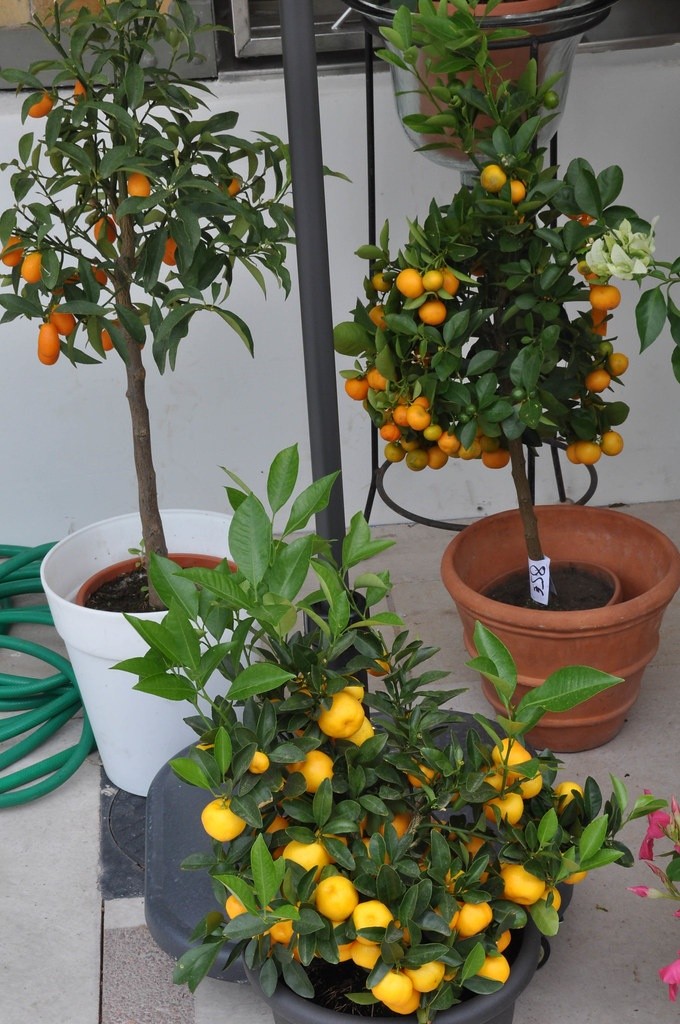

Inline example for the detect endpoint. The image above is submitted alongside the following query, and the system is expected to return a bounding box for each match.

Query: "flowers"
[335,0,680,600]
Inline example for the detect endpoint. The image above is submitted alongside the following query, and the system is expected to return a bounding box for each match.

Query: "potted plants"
[107,441,668,1024]
[0,0,238,614]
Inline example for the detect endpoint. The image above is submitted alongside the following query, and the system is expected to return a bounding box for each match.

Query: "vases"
[479,561,622,606]
[418,0,560,159]
[40,509,264,797]
[439,505,680,753]
